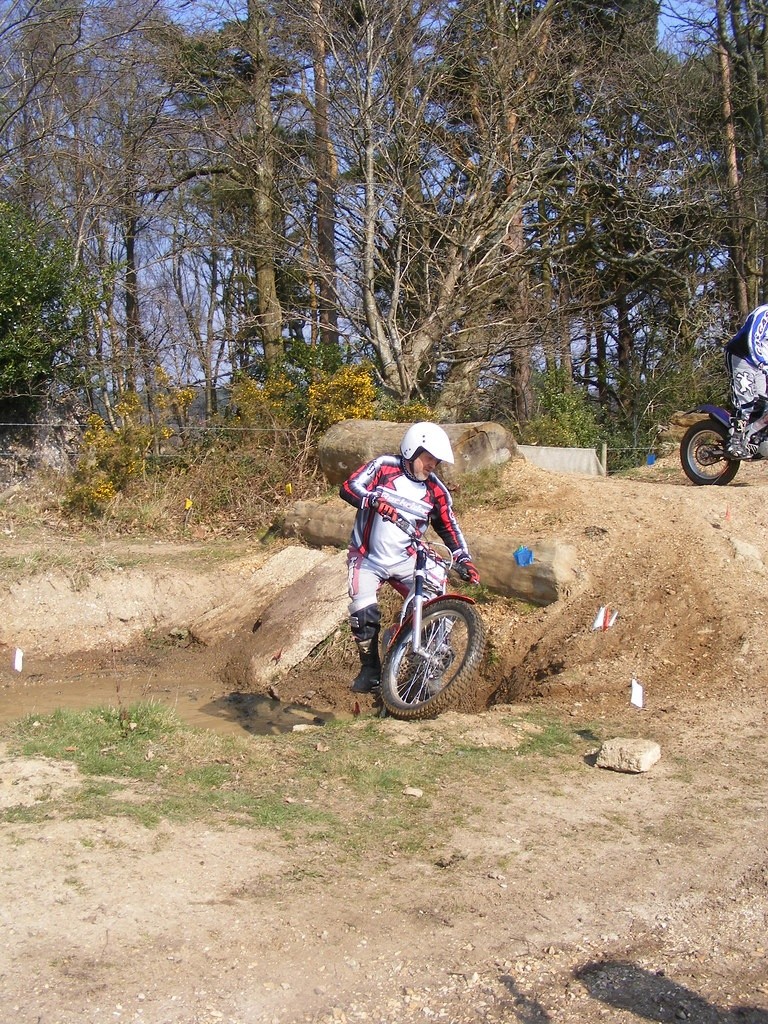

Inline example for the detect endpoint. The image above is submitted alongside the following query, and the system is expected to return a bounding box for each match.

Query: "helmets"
[401,422,457,464]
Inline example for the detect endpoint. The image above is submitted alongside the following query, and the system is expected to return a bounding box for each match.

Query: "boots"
[347,597,381,692]
[428,624,456,672]
[726,419,753,458]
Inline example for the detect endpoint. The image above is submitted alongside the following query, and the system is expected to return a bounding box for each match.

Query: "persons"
[724,304,768,459]
[340,421,480,693]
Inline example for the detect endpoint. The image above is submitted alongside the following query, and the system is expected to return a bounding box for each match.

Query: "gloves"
[376,496,397,522]
[458,562,480,584]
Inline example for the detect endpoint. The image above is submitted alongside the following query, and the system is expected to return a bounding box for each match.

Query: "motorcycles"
[368,494,487,722]
[680,404,768,486]
